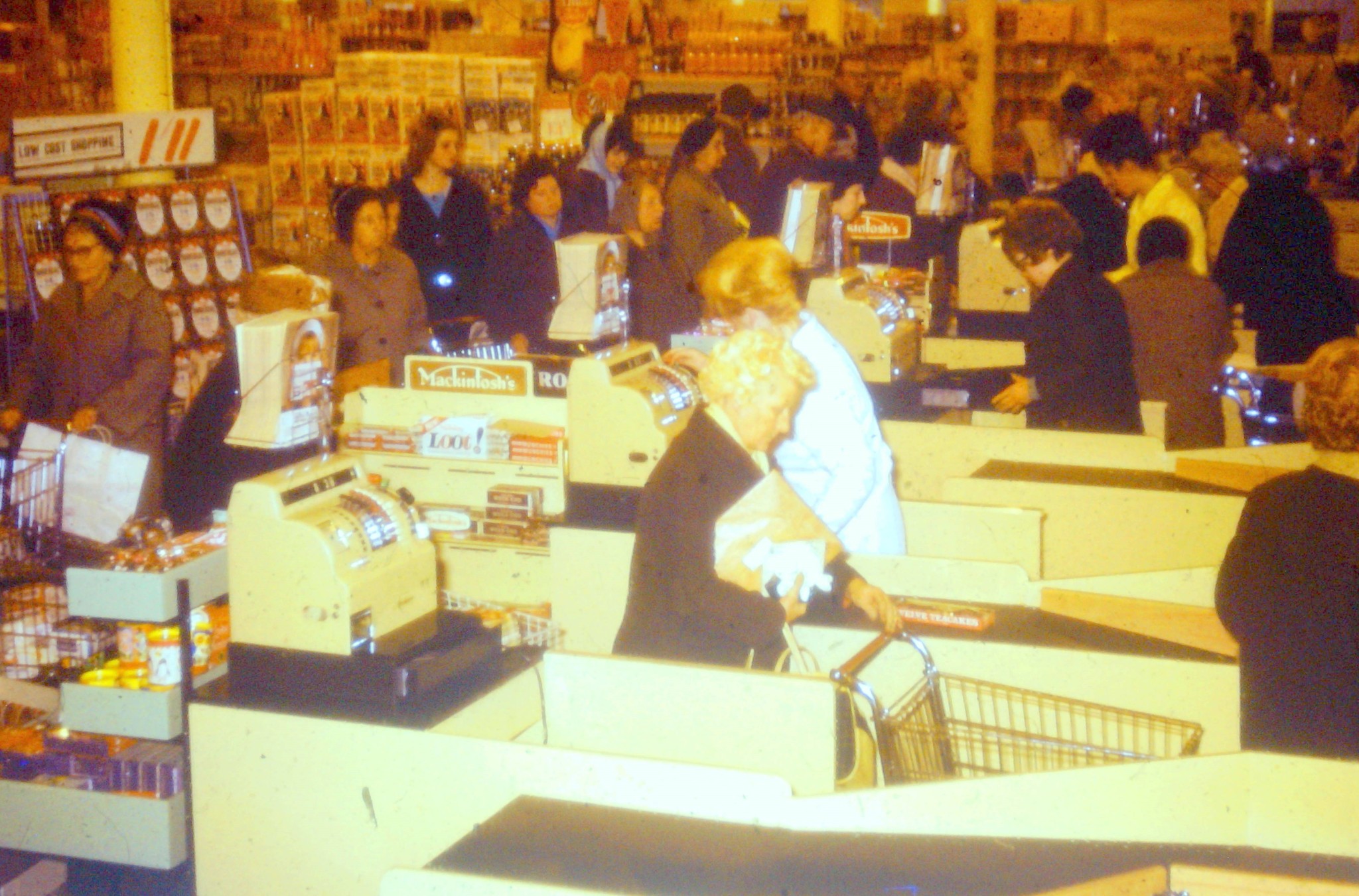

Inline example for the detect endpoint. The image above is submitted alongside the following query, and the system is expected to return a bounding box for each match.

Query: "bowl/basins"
[79,658,148,688]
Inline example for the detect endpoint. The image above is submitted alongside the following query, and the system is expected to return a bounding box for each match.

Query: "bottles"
[501,141,583,213]
[117,597,230,691]
[623,91,717,135]
[684,34,783,74]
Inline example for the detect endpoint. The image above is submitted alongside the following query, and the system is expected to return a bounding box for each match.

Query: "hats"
[800,92,850,139]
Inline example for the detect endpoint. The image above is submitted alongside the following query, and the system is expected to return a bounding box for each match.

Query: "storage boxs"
[477,485,541,541]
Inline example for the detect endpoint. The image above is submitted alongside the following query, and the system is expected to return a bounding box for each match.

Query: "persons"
[612,330,902,675]
[0,196,173,518]
[661,237,904,554]
[301,30,1359,453]
[1212,335,1359,763]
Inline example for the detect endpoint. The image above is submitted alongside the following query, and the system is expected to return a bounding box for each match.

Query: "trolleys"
[1210,366,1314,447]
[830,621,1207,788]
[1,422,76,578]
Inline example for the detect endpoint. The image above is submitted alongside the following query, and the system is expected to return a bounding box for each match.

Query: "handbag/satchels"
[11,422,149,545]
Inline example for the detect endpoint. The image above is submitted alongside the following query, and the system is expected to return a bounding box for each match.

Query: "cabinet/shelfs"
[1,0,1232,316]
[0,530,233,870]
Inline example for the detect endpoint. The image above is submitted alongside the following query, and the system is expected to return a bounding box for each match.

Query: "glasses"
[1012,249,1032,273]
[60,242,107,259]
